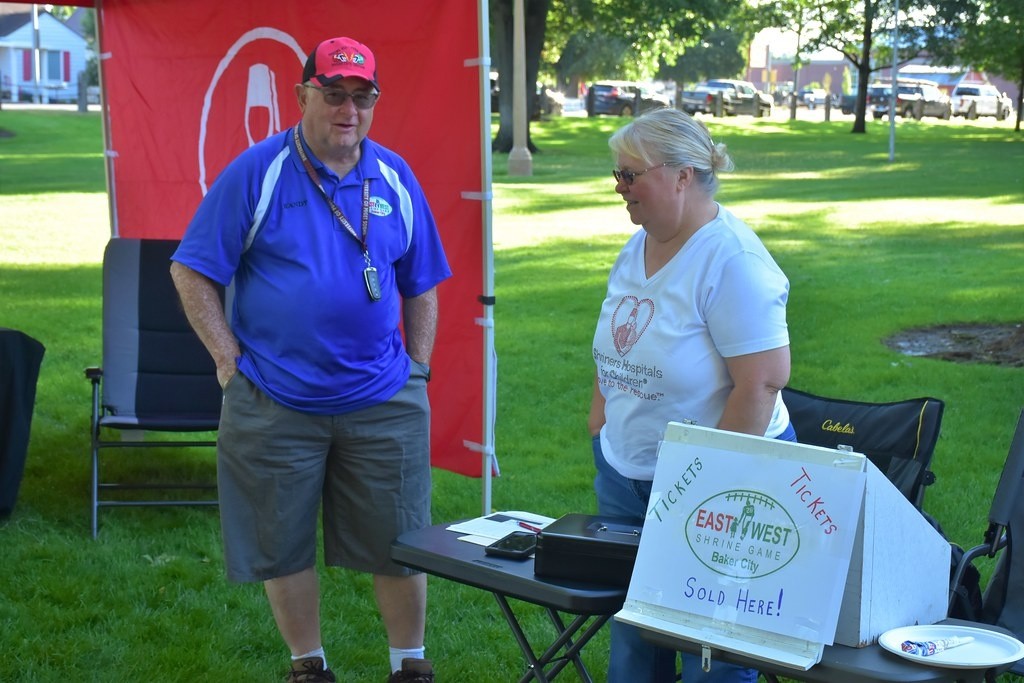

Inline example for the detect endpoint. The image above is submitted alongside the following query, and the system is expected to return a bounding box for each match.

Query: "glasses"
[612,161,668,186]
[301,82,382,109]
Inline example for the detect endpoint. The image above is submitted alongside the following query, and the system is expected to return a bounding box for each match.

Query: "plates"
[877,625,1024,671]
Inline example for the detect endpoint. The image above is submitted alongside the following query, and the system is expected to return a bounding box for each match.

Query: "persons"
[589,107,799,683]
[169,36,454,683]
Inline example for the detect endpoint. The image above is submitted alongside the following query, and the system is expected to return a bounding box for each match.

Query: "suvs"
[952,83,1014,120]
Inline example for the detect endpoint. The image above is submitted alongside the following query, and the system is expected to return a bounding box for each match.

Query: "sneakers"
[288,656,338,682]
[389,657,436,683]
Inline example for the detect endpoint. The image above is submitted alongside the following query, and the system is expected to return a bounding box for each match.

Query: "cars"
[584,80,666,116]
[669,78,951,120]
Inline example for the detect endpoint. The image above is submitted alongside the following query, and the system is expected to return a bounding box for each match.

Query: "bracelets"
[415,362,430,379]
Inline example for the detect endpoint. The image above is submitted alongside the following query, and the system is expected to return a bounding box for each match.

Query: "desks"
[390,513,630,683]
[644,617,1018,683]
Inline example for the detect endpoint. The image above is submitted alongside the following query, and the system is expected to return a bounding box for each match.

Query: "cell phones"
[485,530,538,561]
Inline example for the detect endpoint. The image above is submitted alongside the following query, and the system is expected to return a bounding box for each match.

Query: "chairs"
[781,385,946,508]
[82,236,235,539]
[947,407,1023,676]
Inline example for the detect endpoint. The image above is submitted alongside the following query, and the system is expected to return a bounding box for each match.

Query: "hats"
[300,37,381,92]
